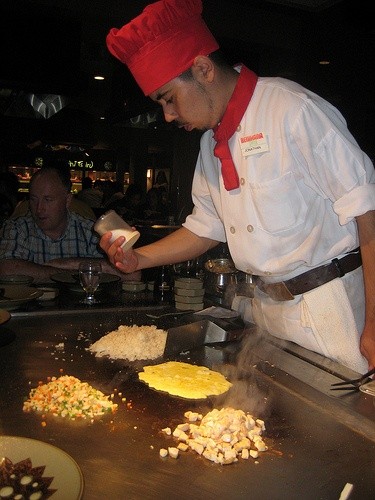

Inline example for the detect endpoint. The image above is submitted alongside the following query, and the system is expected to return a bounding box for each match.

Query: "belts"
[256,247,363,303]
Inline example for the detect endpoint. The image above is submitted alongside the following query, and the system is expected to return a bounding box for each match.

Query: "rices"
[52,325,168,362]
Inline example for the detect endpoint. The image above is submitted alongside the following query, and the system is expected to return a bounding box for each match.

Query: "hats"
[106,0,218,97]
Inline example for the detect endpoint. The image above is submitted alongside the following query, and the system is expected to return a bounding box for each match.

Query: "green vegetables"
[29,384,112,419]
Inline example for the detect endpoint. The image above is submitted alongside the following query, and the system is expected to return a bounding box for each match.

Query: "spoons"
[145,310,194,319]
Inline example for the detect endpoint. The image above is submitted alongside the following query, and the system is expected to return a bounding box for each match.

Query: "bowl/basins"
[203,258,259,296]
[0,270,121,325]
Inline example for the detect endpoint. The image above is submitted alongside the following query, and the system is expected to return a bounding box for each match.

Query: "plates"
[123,281,155,291]
[0,436,84,500]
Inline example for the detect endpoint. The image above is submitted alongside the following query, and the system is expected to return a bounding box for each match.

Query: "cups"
[94,209,140,254]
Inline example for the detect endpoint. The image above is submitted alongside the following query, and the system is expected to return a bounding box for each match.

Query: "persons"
[0,169,141,283]
[147,171,170,206]
[99,180,152,220]
[99,0,375,377]
[78,177,102,204]
[11,164,94,219]
[0,172,27,216]
[168,153,199,223]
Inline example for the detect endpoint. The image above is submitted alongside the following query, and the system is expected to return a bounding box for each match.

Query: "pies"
[138,360,232,399]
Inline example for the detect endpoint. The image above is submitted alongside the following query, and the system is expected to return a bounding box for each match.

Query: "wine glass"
[78,259,102,304]
